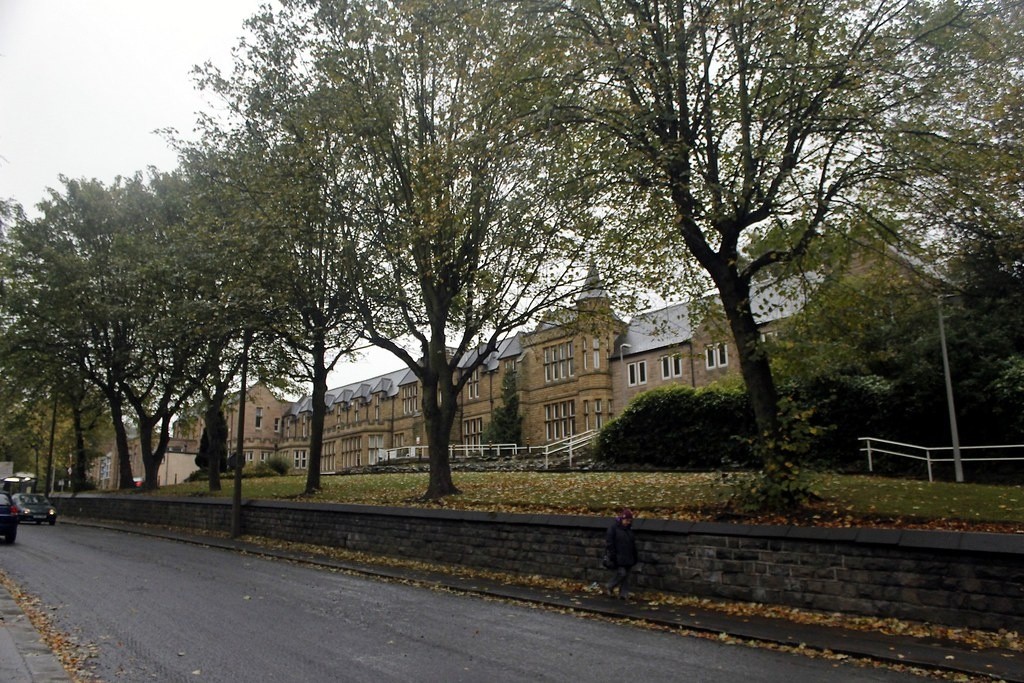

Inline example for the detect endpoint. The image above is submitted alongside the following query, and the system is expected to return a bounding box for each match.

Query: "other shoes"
[605,583,616,597]
[619,592,636,599]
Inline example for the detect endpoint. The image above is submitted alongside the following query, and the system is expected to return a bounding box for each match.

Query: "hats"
[622,509,633,518]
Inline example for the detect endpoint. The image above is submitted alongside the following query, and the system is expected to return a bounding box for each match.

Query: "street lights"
[231,324,255,540]
[937,294,964,482]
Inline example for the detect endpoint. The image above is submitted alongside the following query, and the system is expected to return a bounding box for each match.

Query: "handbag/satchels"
[603,556,614,569]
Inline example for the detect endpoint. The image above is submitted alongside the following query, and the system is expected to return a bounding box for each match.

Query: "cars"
[0,490,20,544]
[11,493,56,525]
